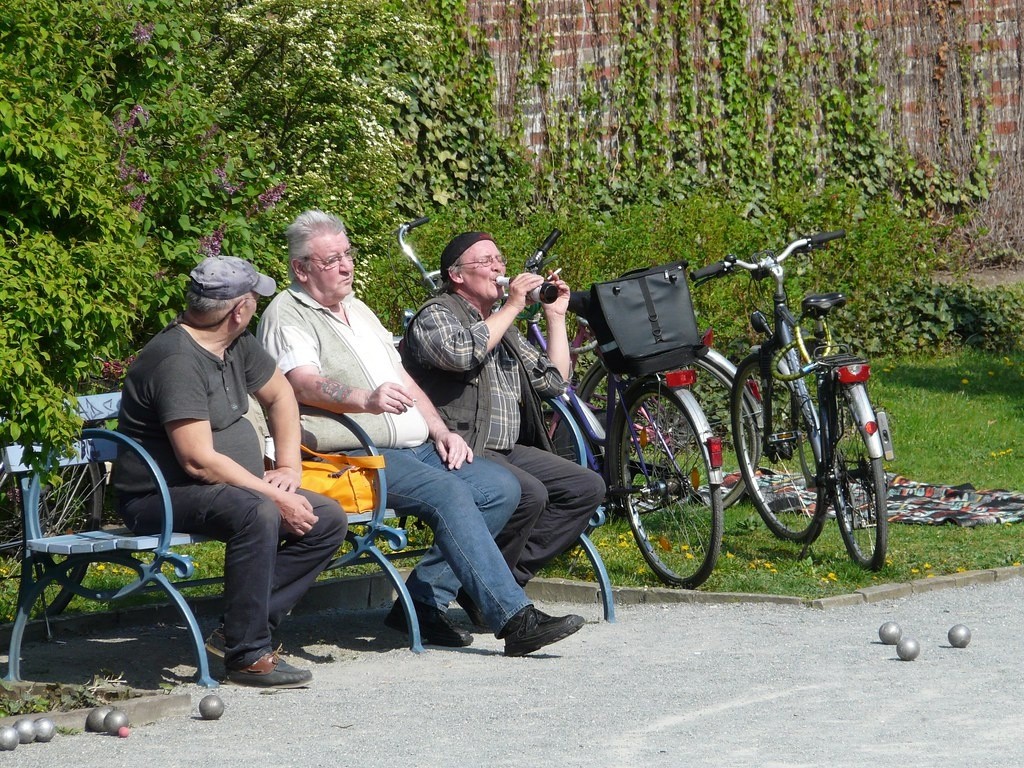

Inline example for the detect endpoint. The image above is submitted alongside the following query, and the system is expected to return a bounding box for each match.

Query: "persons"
[109,256,347,688]
[253,211,586,657]
[403,228,606,632]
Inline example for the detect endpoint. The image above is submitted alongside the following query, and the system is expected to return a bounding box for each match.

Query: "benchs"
[0,390,616,687]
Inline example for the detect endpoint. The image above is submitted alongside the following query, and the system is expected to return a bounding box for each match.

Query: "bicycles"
[686,227,894,572]
[387,212,769,591]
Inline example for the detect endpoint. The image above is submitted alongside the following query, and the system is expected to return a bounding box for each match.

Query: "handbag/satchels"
[300,446,386,513]
[589,262,708,377]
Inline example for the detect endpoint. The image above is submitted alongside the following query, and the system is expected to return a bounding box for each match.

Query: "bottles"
[496,275,559,305]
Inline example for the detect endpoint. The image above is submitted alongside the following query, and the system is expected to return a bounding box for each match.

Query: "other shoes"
[456,590,494,632]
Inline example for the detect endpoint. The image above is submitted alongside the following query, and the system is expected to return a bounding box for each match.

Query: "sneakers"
[204,623,225,657]
[504,606,585,656]
[382,596,473,647]
[227,642,314,689]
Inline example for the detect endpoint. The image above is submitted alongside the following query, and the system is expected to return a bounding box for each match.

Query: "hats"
[440,232,495,282]
[189,255,277,301]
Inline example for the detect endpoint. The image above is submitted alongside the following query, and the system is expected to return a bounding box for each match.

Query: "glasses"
[455,254,507,267]
[305,246,358,268]
